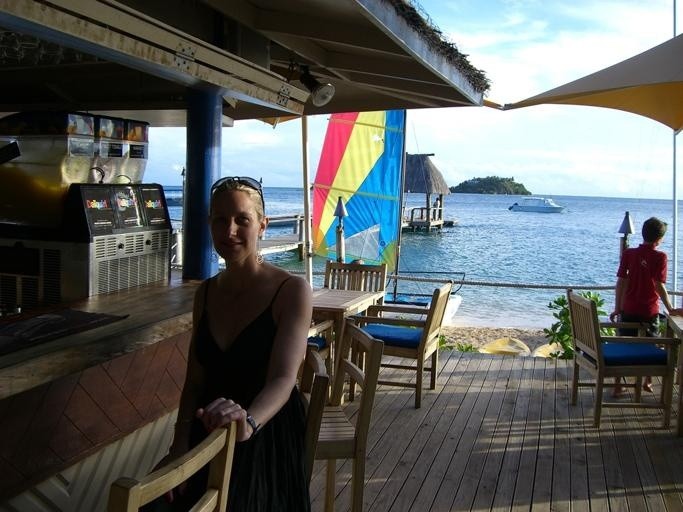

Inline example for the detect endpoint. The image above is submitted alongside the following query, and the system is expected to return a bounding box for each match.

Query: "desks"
[661,298,683,430]
[306,284,385,405]
[2,275,231,512]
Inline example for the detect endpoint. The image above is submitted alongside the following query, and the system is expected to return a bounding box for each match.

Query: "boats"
[508,196,565,213]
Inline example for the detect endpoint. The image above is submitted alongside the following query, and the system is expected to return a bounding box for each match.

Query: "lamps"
[297,72,336,107]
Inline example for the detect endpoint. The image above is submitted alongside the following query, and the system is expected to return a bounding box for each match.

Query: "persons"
[145,179,314,511]
[432,197,441,222]
[609,217,683,397]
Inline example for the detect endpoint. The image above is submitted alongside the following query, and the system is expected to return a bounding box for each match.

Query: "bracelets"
[244,413,262,443]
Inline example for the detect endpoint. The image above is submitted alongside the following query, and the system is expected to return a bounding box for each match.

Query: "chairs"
[292,342,330,474]
[350,278,455,409]
[309,317,385,512]
[324,257,388,290]
[564,286,681,429]
[107,418,238,512]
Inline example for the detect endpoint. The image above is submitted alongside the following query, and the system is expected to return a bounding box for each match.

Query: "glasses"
[210,176,265,216]
